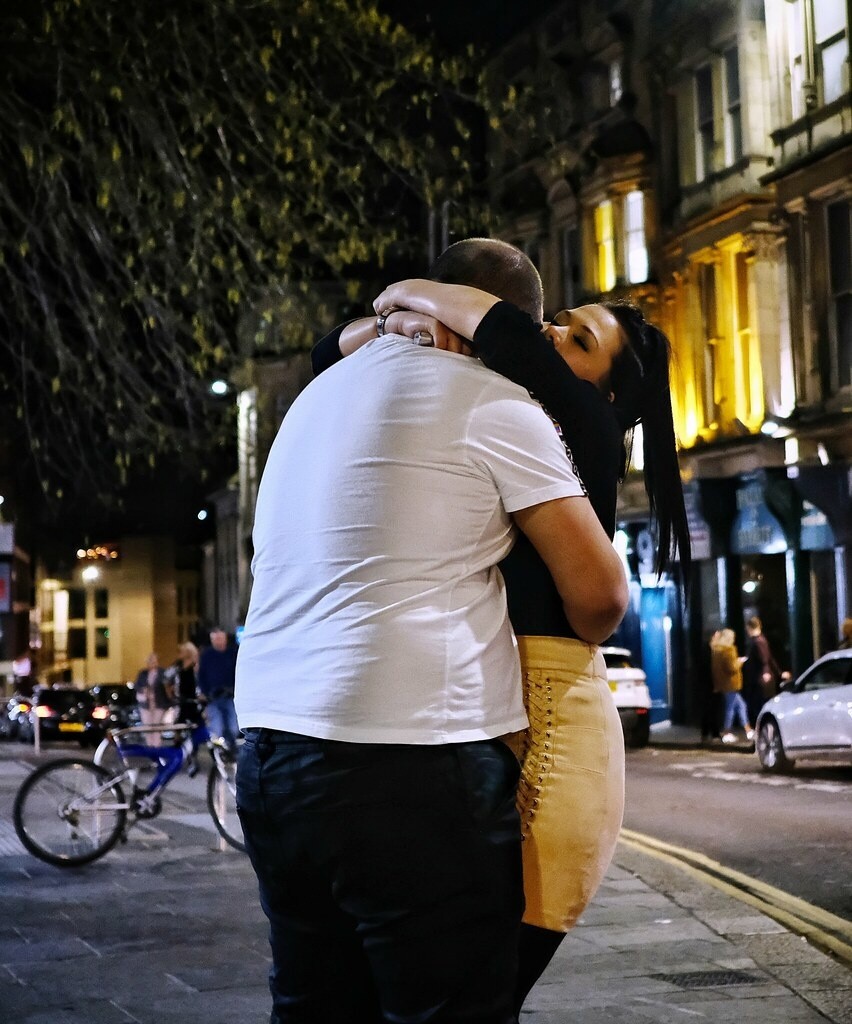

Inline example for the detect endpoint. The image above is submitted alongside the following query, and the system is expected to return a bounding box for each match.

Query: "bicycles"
[13,686,250,867]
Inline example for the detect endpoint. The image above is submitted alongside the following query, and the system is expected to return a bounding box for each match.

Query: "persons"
[232,238,628,1024]
[308,278,691,1024]
[171,641,205,777]
[745,618,789,708]
[197,626,241,759]
[133,654,173,766]
[709,627,757,744]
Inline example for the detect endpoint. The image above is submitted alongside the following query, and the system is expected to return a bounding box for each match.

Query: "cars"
[1,684,146,751]
[753,649,852,778]
[599,645,655,748]
